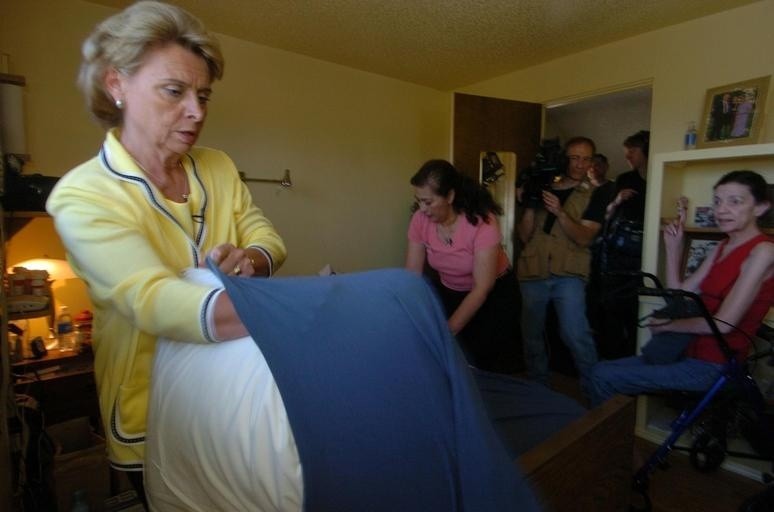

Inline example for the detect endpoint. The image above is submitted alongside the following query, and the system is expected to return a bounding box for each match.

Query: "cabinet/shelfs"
[635,143,774,485]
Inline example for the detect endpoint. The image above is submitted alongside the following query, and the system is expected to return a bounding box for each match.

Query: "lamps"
[238,169,292,187]
[6,255,78,350]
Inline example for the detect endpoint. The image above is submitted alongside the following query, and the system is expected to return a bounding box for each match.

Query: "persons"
[405,159,522,371]
[706,242,718,255]
[43,0,288,512]
[590,169,774,409]
[515,136,602,385]
[587,154,617,197]
[687,247,706,274]
[730,89,755,138]
[604,130,650,355]
[710,93,732,141]
[686,248,697,268]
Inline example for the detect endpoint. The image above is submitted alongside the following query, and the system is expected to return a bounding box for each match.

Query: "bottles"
[56,305,73,353]
[72,310,93,350]
[684,120,696,151]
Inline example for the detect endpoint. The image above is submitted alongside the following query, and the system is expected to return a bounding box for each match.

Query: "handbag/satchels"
[636,299,704,366]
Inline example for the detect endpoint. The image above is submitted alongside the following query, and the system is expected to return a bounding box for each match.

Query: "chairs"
[631,272,774,479]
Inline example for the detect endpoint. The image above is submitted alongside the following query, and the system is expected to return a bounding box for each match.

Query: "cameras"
[516,137,565,203]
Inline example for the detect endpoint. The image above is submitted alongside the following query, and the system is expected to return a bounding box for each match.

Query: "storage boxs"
[43,417,109,512]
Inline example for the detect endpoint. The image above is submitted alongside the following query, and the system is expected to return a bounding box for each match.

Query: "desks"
[0,336,96,512]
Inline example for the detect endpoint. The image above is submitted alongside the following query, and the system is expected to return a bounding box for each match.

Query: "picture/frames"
[689,202,716,228]
[679,233,726,283]
[695,74,773,150]
